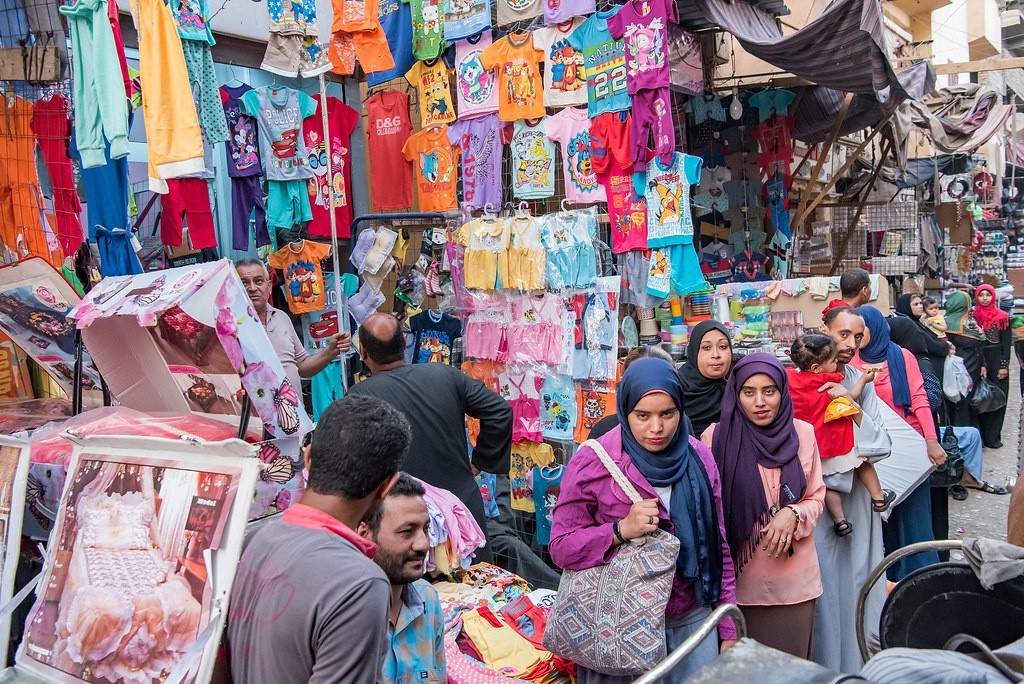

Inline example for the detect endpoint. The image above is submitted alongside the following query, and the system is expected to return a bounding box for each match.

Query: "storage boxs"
[73,262,312,437]
[23,434,306,539]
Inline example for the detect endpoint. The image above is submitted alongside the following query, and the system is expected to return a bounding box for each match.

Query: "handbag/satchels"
[971,376,1007,413]
[943,355,962,403]
[951,353,974,398]
[940,425,964,483]
[542,439,680,677]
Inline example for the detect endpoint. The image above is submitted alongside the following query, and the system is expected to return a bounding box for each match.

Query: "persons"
[355,471,448,684]
[345,312,514,568]
[235,258,351,405]
[227,394,413,684]
[545,269,1012,684]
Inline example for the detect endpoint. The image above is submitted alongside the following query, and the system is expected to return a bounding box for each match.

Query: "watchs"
[613,520,626,543]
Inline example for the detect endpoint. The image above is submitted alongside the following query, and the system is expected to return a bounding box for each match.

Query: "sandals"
[834,520,853,537]
[873,489,896,512]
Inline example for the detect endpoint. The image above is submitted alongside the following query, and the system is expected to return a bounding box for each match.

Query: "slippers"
[951,484,968,500]
[977,480,1007,494]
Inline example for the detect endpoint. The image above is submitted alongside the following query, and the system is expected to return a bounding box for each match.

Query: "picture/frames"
[14,445,261,684]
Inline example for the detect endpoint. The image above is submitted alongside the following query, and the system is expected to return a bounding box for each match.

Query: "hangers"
[0,0,784,473]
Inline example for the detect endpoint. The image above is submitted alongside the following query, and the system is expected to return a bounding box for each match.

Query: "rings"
[781,538,786,542]
[648,516,653,524]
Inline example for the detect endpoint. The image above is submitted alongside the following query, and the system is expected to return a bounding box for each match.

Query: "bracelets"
[785,505,800,522]
